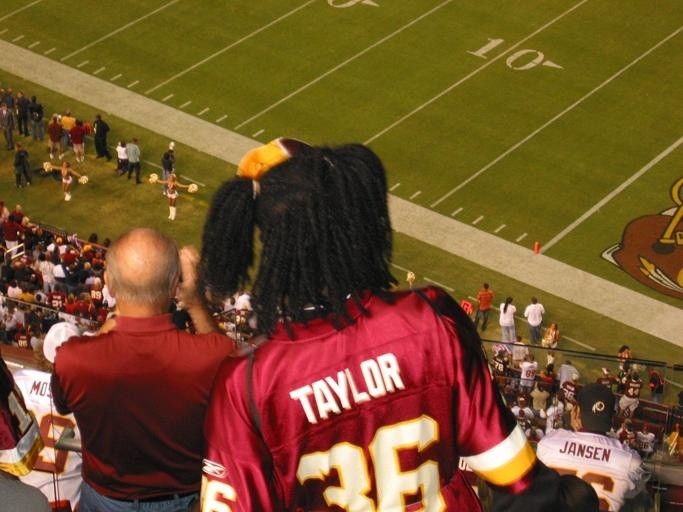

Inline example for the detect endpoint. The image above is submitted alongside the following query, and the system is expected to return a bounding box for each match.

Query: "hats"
[642,423,650,430]
[56,237,63,243]
[519,397,526,402]
[676,424,683,431]
[576,383,616,432]
[43,322,81,364]
[83,245,92,252]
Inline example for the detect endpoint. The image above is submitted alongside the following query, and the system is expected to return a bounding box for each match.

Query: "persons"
[126,139,143,184]
[52,229,236,512]
[0,103,13,150]
[537,383,653,512]
[542,323,560,358]
[156,175,189,221]
[666,390,683,434]
[61,110,77,150]
[0,205,116,352]
[162,152,173,196]
[12,144,31,188]
[198,138,546,511]
[491,339,577,444]
[524,297,545,342]
[636,426,657,459]
[664,422,683,460]
[474,283,495,332]
[618,363,628,392]
[599,368,617,388]
[616,420,639,451]
[47,117,64,160]
[0,87,14,108]
[499,298,517,343]
[618,347,629,370]
[92,116,112,162]
[617,371,643,418]
[15,90,31,137]
[29,96,44,142]
[170,291,196,337]
[648,366,665,402]
[70,120,85,163]
[115,141,128,175]
[50,163,80,200]
[204,290,260,345]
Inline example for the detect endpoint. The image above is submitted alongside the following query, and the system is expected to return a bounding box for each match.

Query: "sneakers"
[128,176,131,179]
[163,191,166,195]
[59,153,64,160]
[96,155,104,159]
[76,158,80,162]
[107,156,112,162]
[65,195,67,200]
[67,195,71,201]
[81,156,84,162]
[50,154,54,159]
[40,138,43,140]
[33,138,37,140]
[136,181,144,183]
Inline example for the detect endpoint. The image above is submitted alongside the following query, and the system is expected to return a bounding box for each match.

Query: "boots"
[168,206,171,219]
[171,207,176,220]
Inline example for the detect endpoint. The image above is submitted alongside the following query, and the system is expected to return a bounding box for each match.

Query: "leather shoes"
[19,131,24,135]
[4,145,8,147]
[25,132,32,136]
[8,147,14,150]
